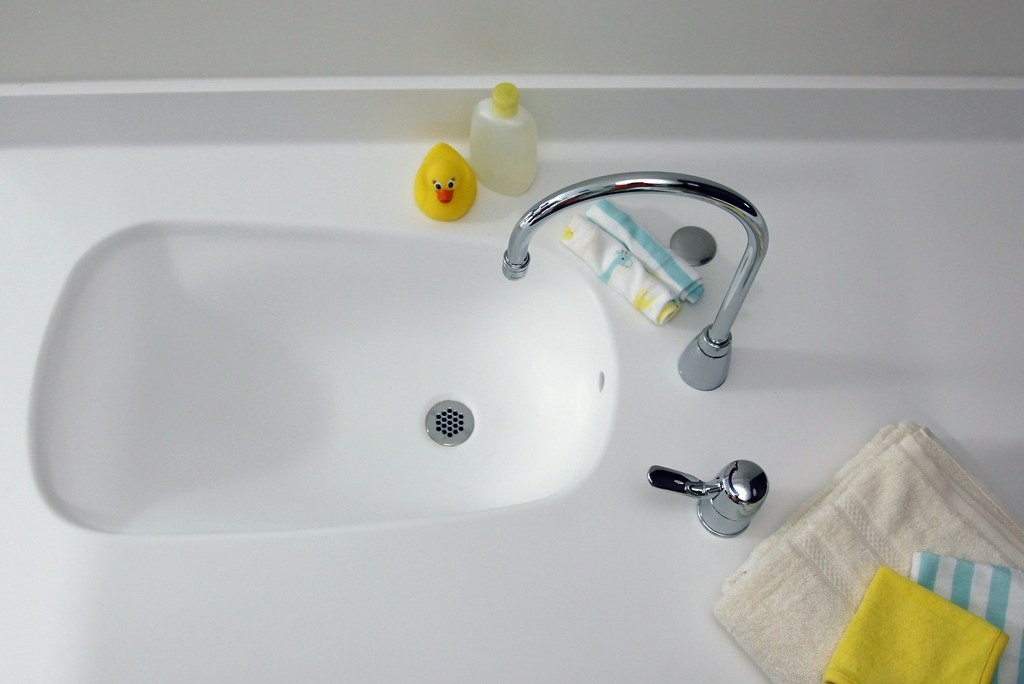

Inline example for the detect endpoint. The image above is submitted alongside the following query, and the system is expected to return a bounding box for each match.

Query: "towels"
[713,418,1024,684]
[557,213,682,327]
[820,564,1010,684]
[907,550,1024,684]
[584,197,706,304]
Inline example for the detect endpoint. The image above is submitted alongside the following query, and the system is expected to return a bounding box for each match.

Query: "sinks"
[24,213,620,541]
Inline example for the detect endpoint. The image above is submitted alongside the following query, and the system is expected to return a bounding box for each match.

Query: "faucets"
[501,170,769,392]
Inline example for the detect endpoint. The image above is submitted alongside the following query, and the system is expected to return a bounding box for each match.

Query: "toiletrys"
[468,81,538,196]
